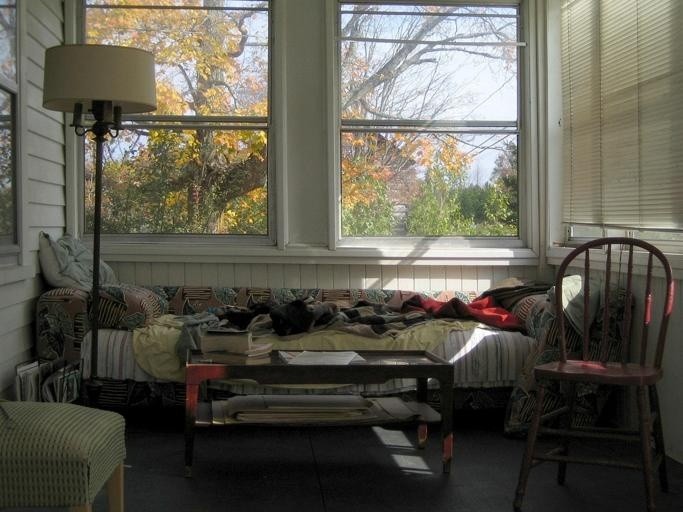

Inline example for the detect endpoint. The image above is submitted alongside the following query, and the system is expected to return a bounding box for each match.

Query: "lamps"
[44,41,158,409]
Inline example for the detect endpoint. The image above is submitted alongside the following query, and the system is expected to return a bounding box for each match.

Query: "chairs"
[513,236,676,512]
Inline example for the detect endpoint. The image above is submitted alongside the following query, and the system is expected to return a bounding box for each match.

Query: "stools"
[1,398,128,511]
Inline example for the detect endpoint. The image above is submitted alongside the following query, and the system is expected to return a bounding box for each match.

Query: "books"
[199,327,274,366]
[209,394,379,425]
[278,349,366,365]
[12,360,81,404]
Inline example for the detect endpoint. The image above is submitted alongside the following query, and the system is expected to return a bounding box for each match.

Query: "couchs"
[30,275,640,439]
